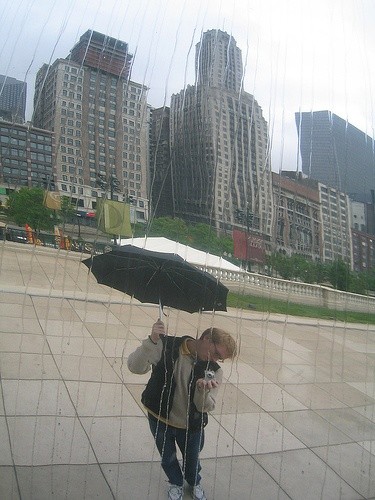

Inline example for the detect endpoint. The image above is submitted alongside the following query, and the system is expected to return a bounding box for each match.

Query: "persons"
[126,315,239,500]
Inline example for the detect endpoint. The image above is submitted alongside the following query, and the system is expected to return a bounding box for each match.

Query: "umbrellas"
[81,242,228,328]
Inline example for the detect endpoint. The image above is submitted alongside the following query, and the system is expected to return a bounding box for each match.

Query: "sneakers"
[168,483,184,500]
[191,485,206,500]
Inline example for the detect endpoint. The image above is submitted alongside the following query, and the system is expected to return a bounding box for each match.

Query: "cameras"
[204,370,216,385]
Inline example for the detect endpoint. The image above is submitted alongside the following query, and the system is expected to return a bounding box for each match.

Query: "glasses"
[212,343,224,362]
